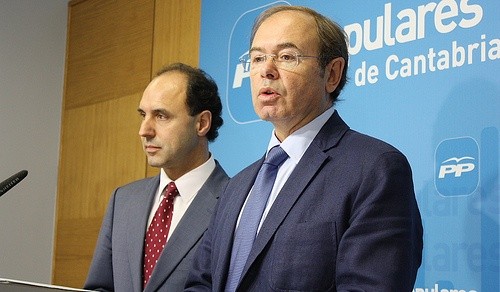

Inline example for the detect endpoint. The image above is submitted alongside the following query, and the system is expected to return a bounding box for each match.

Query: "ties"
[140,182,178,292]
[222,147,289,292]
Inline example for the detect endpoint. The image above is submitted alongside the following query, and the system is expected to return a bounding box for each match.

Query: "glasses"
[239,50,322,71]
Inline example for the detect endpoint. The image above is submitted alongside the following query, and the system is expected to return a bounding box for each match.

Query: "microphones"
[0,170,28,197]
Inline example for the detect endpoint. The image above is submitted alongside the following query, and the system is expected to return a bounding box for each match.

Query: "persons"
[84,63,231,292]
[184,5,423,292]
